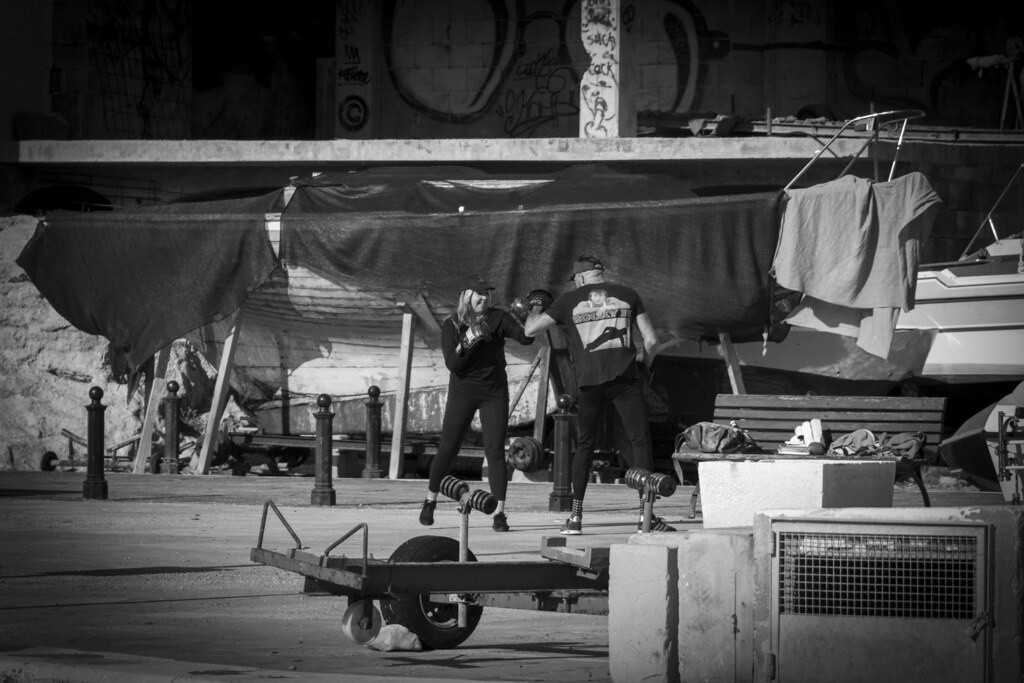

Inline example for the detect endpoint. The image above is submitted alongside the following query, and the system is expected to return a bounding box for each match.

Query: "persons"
[523,254,661,535]
[417,273,536,532]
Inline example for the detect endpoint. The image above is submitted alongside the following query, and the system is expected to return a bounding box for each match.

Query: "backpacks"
[681,421,764,455]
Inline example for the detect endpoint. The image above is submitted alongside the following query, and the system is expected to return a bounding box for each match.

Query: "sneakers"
[419,497,437,526]
[492,511,510,532]
[559,515,582,535]
[638,515,677,533]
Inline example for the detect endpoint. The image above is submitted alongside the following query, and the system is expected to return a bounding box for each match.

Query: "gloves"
[461,320,492,353]
[637,361,654,396]
[510,294,531,323]
[525,289,555,312]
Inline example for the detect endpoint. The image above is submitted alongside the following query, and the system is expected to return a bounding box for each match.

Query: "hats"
[568,255,606,281]
[458,274,496,294]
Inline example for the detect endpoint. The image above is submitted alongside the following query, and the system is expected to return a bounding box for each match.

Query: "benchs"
[671,393,947,518]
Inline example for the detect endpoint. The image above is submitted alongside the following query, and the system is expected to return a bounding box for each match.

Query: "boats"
[40,73,1024,436]
